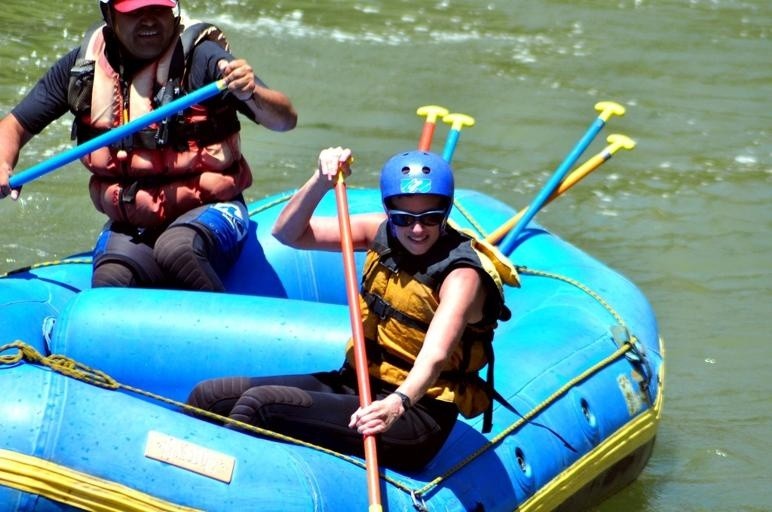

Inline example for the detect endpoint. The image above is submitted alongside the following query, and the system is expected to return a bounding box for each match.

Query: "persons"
[0,1,296,296]
[180,141,517,474]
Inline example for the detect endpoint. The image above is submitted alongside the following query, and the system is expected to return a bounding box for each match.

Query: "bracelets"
[242,91,256,102]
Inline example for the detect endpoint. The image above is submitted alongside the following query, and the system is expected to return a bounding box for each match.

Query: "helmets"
[381,149,455,233]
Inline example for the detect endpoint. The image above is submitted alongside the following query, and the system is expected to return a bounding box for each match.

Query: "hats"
[113,0,178,14]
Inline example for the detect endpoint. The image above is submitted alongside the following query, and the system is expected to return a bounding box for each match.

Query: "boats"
[1,183,668,512]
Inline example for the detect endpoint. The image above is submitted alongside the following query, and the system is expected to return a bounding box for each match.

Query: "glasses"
[386,208,444,226]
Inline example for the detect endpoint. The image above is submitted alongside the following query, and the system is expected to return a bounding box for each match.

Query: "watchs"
[392,391,410,412]
